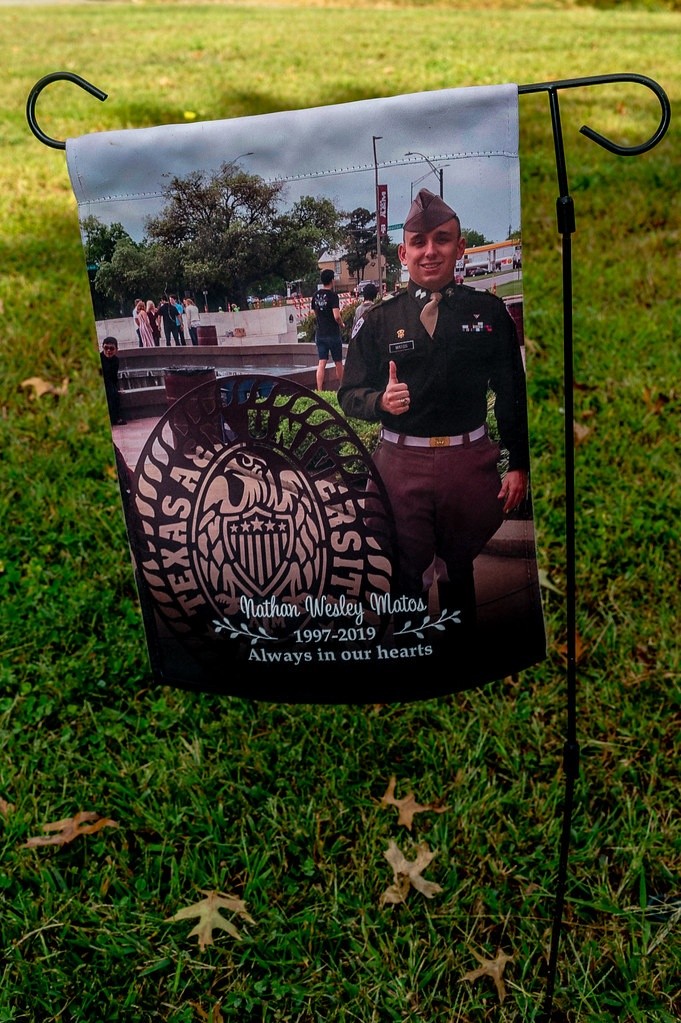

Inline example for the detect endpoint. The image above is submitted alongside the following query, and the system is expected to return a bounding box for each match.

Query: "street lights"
[411,164,450,205]
[404,152,444,201]
[372,135,384,302]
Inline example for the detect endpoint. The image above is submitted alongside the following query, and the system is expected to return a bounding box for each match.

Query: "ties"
[418,292,443,337]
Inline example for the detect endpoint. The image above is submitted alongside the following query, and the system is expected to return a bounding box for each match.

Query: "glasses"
[102,346,117,351]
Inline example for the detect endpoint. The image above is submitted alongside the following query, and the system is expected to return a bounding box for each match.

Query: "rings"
[401,398,407,406]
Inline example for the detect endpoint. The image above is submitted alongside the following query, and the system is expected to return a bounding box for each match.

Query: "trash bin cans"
[163,368,222,452]
[196,325,218,345]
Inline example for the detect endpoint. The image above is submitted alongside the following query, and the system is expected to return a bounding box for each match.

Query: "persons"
[99,336,128,425]
[312,269,346,392]
[336,188,529,642]
[352,284,377,329]
[132,294,200,348]
[231,304,240,312]
[218,307,223,312]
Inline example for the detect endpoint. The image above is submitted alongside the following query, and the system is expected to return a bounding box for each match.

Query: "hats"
[402,187,456,234]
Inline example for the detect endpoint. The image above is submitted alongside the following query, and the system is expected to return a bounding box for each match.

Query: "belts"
[381,424,485,448]
